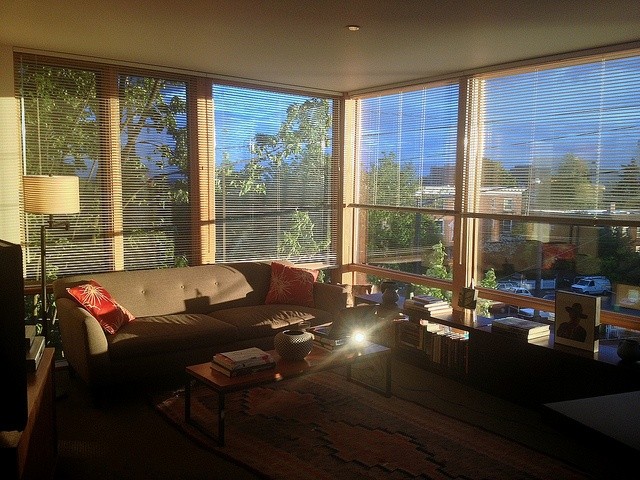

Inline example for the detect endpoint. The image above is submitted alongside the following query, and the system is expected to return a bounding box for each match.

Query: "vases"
[272,329,315,361]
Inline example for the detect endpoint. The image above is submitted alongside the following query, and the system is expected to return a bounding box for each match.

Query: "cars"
[495,283,514,291]
[488,300,518,314]
[517,307,555,324]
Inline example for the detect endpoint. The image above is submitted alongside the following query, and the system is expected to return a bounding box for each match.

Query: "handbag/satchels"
[458,278,479,310]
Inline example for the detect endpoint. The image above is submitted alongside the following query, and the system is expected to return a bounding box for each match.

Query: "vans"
[571,276,613,296]
[507,287,533,298]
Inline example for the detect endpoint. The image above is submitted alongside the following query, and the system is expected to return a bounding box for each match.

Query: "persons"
[558,304,589,341]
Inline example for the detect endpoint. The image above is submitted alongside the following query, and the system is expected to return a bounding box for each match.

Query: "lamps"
[23,174,80,348]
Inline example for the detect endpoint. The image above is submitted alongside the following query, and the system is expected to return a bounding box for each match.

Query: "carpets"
[156,370,596,476]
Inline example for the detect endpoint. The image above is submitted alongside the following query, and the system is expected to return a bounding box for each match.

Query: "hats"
[565,303,588,320]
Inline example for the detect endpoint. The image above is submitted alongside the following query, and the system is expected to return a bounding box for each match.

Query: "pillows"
[66,279,136,335]
[265,262,319,308]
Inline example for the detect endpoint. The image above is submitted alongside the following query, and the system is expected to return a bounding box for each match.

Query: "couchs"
[53,259,354,389]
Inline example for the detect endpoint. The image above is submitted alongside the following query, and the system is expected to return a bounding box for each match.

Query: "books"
[210,346,277,376]
[395,323,470,376]
[402,293,452,317]
[492,316,551,345]
[306,329,346,352]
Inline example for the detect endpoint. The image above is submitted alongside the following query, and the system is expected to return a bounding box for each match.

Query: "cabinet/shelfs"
[355,293,640,397]
[0,347,57,480]
